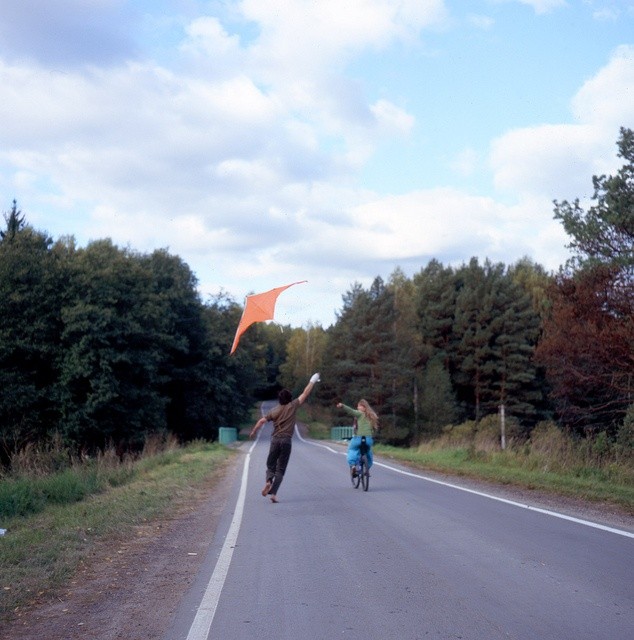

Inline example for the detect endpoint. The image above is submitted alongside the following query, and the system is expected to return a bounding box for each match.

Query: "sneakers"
[352,466,356,478]
[364,471,370,477]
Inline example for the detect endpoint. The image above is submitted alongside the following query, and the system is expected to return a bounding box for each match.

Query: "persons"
[249,373,319,502]
[336,399,379,477]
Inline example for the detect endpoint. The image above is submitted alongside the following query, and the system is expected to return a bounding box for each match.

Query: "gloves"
[310,373,319,383]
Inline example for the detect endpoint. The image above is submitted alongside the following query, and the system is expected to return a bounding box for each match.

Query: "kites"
[229,281,308,355]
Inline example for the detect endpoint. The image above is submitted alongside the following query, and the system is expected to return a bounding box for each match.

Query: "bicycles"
[341,436,370,492]
